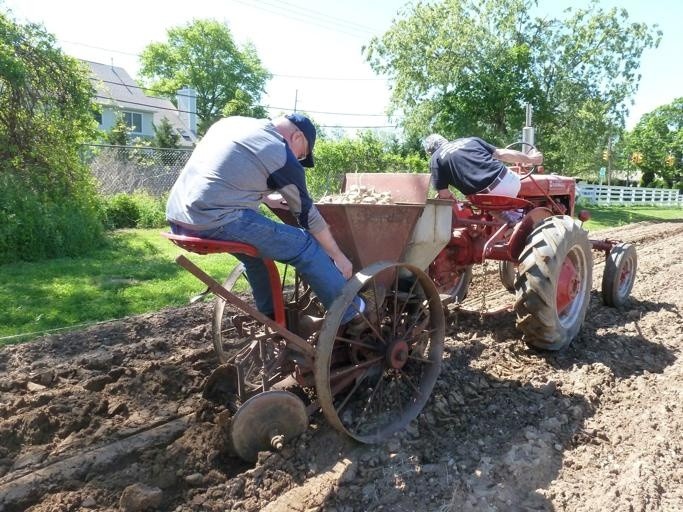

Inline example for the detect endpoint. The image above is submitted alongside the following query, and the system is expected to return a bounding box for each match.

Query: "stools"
[160,230,288,331]
[464,193,536,212]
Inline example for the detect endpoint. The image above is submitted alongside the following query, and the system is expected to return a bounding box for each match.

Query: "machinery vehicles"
[156,99,640,467]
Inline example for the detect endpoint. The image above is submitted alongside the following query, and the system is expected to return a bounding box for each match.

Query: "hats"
[285,114,316,168]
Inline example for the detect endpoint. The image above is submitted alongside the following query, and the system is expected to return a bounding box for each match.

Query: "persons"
[162,112,387,343]
[422,132,546,225]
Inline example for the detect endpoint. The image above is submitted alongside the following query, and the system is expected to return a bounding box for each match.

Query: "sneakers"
[349,284,386,325]
[267,308,304,322]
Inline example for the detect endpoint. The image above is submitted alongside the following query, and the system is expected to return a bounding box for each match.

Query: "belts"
[479,165,508,194]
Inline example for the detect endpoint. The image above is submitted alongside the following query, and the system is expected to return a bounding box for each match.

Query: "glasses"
[295,128,307,162]
